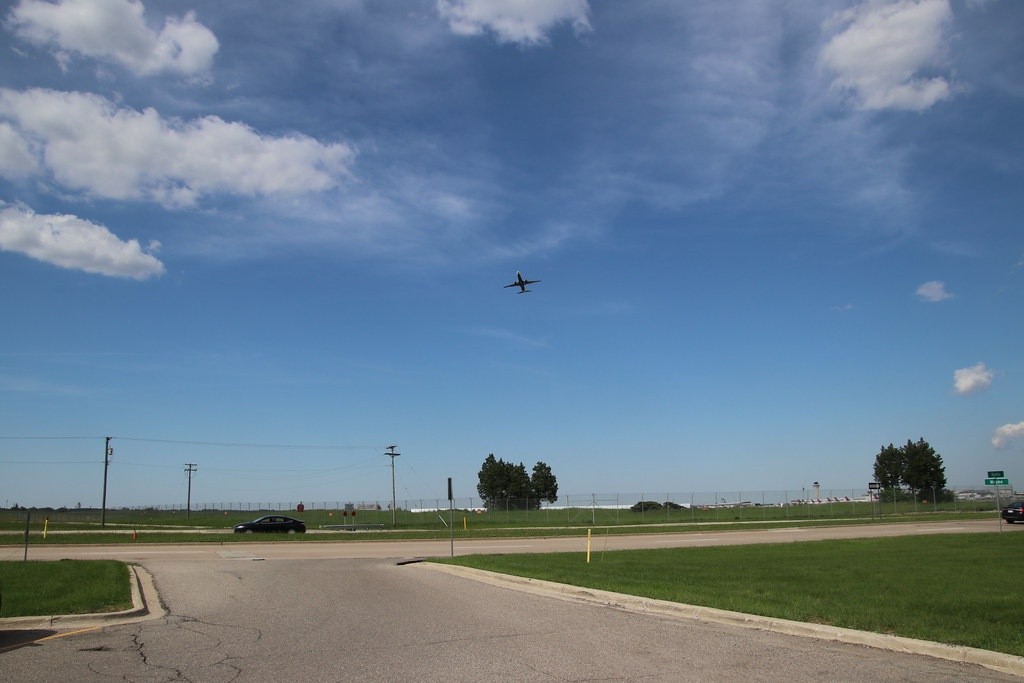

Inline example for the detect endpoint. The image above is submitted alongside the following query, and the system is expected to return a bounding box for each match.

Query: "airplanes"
[503,269,540,294]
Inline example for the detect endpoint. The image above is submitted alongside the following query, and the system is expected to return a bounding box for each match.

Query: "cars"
[1001,500,1024,524]
[233,516,307,535]
